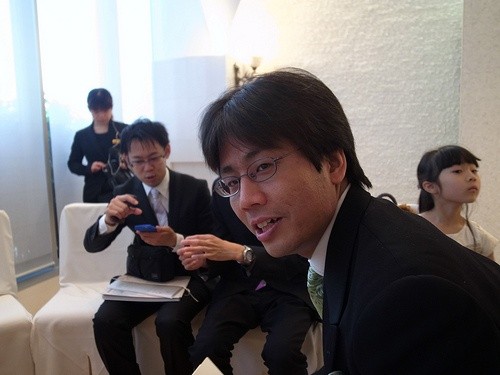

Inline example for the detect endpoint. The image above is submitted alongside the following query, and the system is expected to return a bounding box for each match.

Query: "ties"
[149,188,168,226]
[307,267,323,318]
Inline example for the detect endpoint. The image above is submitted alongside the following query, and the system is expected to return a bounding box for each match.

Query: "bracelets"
[104,220,118,226]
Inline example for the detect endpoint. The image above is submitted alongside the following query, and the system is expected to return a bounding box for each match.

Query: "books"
[102,277,189,301]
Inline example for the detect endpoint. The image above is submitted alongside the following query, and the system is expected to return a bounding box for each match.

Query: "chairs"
[0,203,325,375]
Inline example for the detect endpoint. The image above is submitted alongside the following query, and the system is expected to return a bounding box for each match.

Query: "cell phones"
[134,224,156,233]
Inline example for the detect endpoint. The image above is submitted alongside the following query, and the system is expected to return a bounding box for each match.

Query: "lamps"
[233,55,261,88]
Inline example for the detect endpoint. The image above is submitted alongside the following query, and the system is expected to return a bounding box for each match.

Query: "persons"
[196,67,500,375]
[67,88,322,375]
[416,146,500,264]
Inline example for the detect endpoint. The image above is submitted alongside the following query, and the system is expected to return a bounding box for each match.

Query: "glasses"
[213,147,299,197]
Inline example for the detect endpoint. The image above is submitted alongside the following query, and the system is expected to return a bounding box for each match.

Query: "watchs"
[237,245,253,265]
